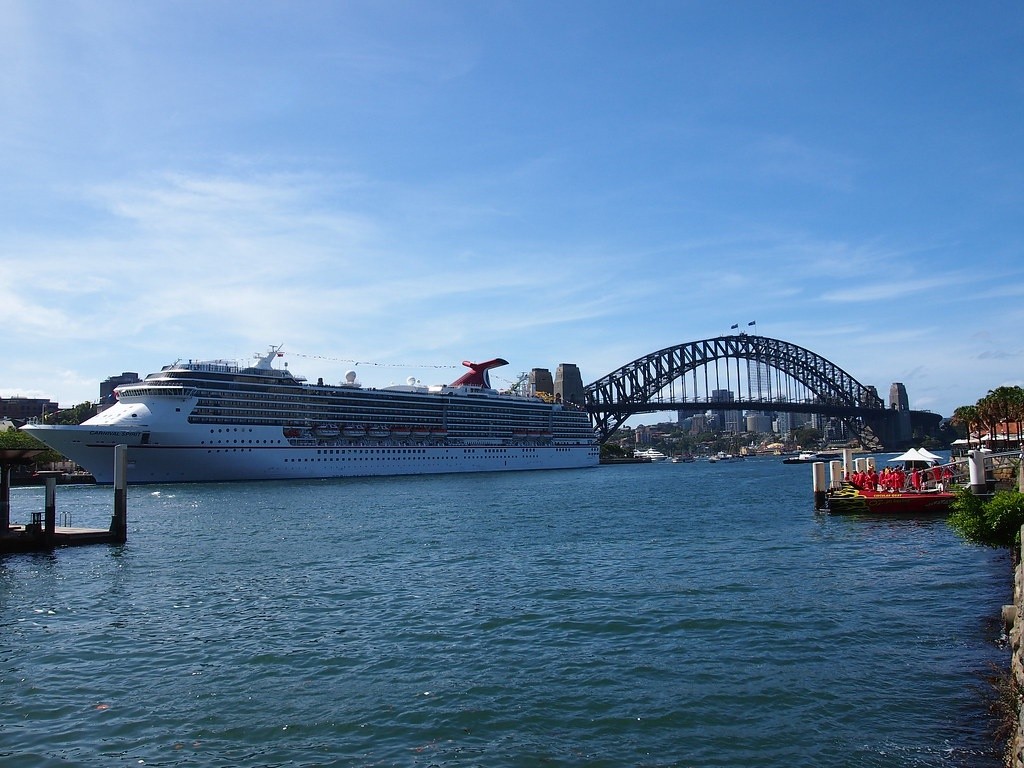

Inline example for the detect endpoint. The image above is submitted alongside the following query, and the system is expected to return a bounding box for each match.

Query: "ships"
[17,340,601,485]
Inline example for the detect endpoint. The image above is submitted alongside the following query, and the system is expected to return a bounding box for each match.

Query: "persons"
[950,456,958,470]
[845,463,907,493]
[920,469,929,490]
[933,460,939,467]
[911,468,921,491]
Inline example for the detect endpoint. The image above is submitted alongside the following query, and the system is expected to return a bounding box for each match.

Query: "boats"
[633,447,668,464]
[673,450,745,463]
[770,445,843,465]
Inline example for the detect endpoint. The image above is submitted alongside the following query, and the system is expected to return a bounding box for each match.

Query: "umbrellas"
[885,447,944,472]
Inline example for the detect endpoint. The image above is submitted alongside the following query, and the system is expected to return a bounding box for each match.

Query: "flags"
[277,354,283,357]
[747,320,756,326]
[731,323,738,329]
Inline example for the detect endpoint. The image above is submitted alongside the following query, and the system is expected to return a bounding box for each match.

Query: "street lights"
[42,404,46,425]
